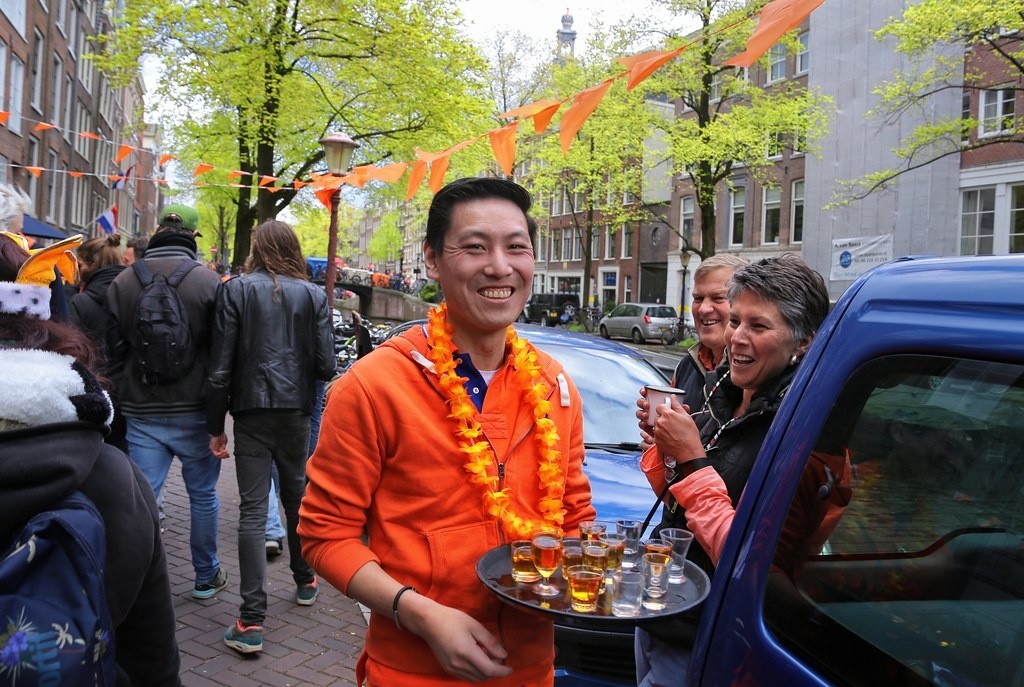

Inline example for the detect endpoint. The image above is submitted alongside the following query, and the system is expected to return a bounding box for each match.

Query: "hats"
[158,204,203,239]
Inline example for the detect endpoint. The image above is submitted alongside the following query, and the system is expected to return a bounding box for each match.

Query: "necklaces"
[425,300,568,544]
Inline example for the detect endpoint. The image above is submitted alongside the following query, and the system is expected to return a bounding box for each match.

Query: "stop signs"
[210,246,218,254]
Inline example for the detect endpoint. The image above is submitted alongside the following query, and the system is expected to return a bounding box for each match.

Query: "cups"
[616,519,644,568]
[611,571,646,617]
[559,536,586,580]
[657,527,694,584]
[565,565,606,613]
[641,553,674,610]
[597,532,627,585]
[578,521,606,542]
[511,539,543,583]
[642,538,674,563]
[645,384,686,428]
[581,539,610,576]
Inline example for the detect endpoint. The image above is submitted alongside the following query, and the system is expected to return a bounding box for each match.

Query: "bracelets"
[392,586,420,631]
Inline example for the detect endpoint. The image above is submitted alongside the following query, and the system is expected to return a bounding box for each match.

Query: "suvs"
[516,293,581,328]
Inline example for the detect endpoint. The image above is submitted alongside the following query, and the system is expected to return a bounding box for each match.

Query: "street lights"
[318,127,360,318]
[677,250,691,341]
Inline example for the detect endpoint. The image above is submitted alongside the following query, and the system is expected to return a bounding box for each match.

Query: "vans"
[599,302,677,344]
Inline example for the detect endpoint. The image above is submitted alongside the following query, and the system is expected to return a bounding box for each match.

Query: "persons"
[672,252,753,413]
[204,219,336,654]
[633,252,856,686]
[102,203,230,599]
[0,184,335,687]
[295,176,596,687]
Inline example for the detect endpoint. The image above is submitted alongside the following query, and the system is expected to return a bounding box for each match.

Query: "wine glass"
[529,528,561,596]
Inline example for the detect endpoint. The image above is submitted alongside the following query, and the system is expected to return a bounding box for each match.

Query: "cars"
[687,255,1024,686]
[380,319,672,687]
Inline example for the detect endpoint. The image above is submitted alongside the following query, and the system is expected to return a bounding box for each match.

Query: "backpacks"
[130,261,200,379]
[0,486,113,687]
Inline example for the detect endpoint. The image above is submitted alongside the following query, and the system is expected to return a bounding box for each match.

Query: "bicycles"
[661,317,700,348]
[583,305,602,333]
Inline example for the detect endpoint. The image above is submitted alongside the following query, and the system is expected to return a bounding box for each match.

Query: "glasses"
[78,261,93,270]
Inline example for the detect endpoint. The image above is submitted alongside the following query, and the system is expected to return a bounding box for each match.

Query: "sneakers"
[264,539,283,554]
[297,575,319,604]
[192,567,228,598]
[223,619,263,652]
